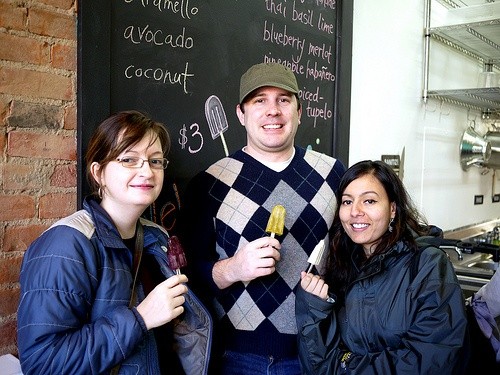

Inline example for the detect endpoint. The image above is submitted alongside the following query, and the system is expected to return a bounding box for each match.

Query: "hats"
[239,62,298,103]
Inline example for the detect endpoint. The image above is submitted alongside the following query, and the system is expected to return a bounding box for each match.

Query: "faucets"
[440,244,464,263]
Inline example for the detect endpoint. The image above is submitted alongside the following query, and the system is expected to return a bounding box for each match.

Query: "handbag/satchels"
[407,244,500,375]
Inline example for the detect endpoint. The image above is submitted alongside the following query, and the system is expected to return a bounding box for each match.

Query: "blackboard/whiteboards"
[76,0,354,226]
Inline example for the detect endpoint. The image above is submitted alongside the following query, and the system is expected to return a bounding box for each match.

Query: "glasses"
[114,156,169,170]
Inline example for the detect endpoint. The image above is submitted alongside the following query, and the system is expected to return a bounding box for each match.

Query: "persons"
[16,111,212,375]
[294,159,468,375]
[178,62,346,375]
